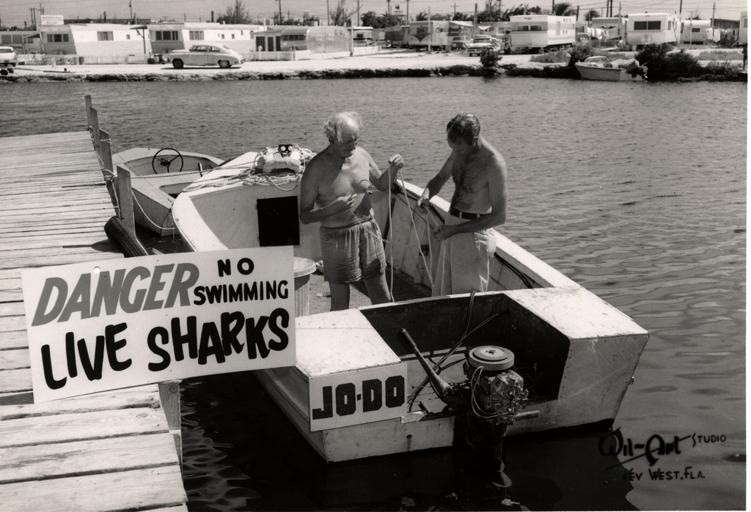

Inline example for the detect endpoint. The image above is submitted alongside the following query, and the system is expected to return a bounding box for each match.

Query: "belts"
[447,206,491,221]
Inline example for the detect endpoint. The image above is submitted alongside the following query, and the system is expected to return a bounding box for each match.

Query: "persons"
[411,111,509,299]
[740,43,747,70]
[628,55,649,79]
[297,108,405,315]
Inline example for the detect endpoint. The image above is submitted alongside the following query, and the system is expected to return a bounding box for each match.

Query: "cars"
[466,31,506,60]
[168,42,245,69]
[0,45,18,67]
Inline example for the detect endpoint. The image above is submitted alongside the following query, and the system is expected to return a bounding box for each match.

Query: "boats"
[169,144,651,466]
[111,142,224,237]
